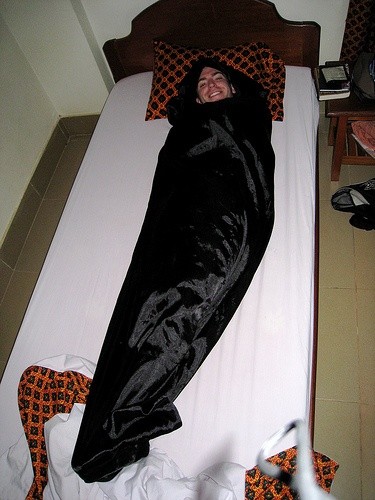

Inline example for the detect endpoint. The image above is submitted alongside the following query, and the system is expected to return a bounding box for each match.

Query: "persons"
[72,54,277,486]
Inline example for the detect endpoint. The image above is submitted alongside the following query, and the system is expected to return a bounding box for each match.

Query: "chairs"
[325,99,374,181]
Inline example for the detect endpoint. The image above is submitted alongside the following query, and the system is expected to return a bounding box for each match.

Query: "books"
[313,60,351,102]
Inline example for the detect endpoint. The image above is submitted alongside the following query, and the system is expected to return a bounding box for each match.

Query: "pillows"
[144,38,287,122]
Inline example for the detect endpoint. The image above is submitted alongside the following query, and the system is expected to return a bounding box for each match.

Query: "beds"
[0,62,320,500]
